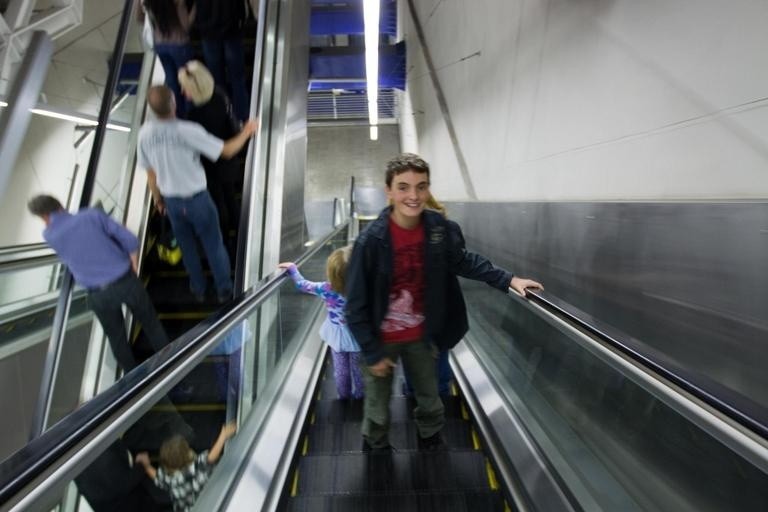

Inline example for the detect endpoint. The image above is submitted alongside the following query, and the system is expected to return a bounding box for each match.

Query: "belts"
[87,272,130,292]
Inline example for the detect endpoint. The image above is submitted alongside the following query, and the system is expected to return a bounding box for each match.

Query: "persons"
[136,84,259,303]
[197,0,258,123]
[386,191,471,396]
[134,421,237,511]
[177,58,242,243]
[28,194,200,400]
[134,0,196,118]
[277,246,364,420]
[343,153,546,455]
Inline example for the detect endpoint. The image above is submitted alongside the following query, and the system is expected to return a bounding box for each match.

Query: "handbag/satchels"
[157,232,184,273]
